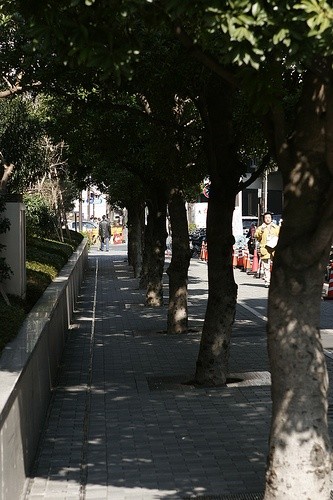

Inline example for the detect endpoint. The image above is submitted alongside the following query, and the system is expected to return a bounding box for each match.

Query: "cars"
[72,221,97,231]
[189,227,208,258]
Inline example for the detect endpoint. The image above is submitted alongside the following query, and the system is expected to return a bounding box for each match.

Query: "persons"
[98,215,111,253]
[254,212,280,289]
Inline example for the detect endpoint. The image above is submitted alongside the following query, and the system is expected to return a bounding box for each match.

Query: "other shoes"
[265,283,269,288]
[106,250,108,251]
[100,249,103,251]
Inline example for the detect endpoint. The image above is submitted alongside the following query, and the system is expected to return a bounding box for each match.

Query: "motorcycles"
[246,230,257,255]
